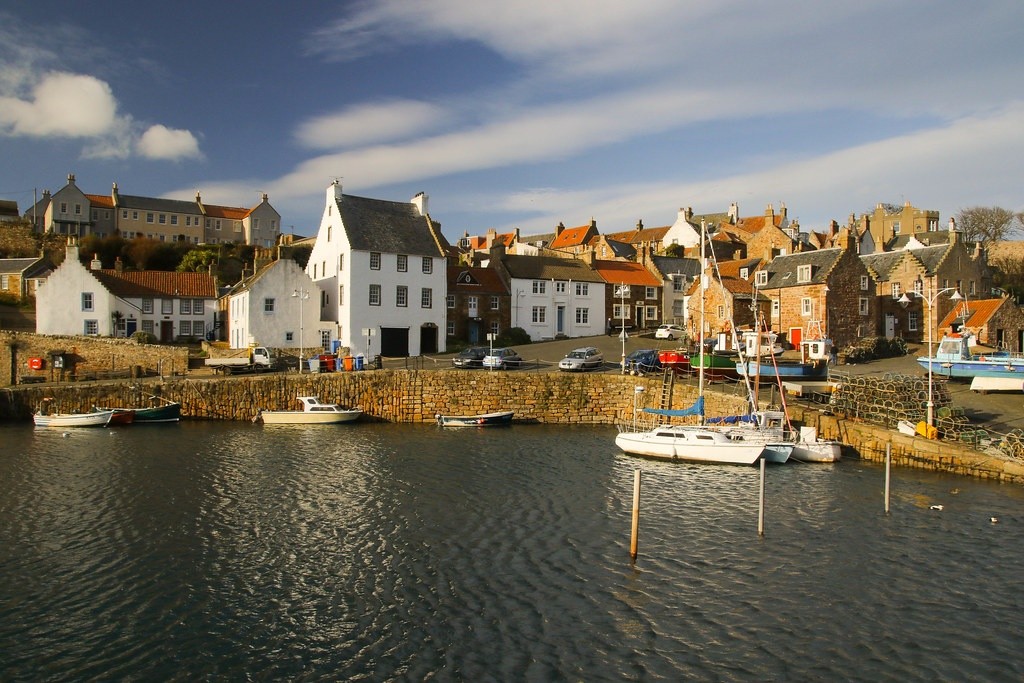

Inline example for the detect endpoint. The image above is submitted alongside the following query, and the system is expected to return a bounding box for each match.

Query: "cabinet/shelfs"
[319,355,337,372]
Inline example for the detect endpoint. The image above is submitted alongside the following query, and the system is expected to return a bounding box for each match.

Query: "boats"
[968,376,1024,397]
[260,395,365,425]
[33,397,116,429]
[111,410,136,428]
[96,402,181,426]
[915,332,1024,378]
[623,304,842,465]
[435,411,514,430]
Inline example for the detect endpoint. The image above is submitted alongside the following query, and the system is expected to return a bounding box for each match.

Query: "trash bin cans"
[355,357,364,370]
[308,355,319,367]
[374,355,382,370]
[343,357,353,371]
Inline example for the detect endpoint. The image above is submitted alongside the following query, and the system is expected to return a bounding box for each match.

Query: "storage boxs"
[898,421,917,436]
[659,352,684,362]
[915,421,938,439]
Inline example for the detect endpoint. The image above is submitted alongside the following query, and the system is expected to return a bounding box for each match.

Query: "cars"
[483,348,522,370]
[655,324,688,340]
[452,347,491,368]
[558,348,604,373]
[620,349,662,374]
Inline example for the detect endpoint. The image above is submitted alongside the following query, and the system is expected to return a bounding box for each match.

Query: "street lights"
[615,280,630,376]
[292,282,310,374]
[897,287,964,437]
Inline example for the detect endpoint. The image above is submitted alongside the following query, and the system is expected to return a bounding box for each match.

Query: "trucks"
[204,346,276,376]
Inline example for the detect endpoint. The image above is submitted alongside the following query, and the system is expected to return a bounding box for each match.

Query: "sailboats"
[616,217,767,466]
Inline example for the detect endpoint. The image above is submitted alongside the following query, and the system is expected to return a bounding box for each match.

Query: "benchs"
[20,376,47,384]
